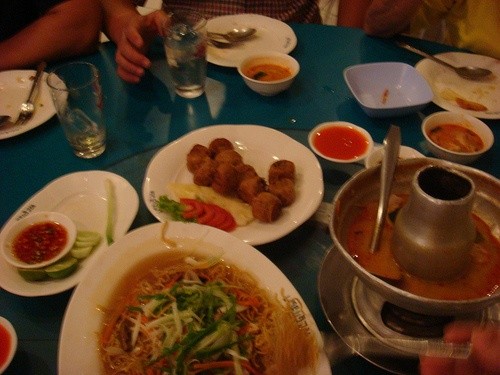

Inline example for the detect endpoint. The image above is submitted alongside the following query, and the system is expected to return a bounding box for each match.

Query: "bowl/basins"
[343,62,433,118]
[237,51,301,97]
[422,111,494,165]
[1,212,77,269]
[308,121,374,163]
[0,315,18,375]
[366,144,425,170]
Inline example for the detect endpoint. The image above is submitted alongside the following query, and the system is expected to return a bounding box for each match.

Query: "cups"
[46,62,107,160]
[162,9,208,100]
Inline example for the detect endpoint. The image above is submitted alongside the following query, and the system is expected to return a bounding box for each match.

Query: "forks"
[13,61,47,126]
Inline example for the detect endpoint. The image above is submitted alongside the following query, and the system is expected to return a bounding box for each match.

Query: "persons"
[0,0,100,70]
[363,1,500,60]
[101,0,323,82]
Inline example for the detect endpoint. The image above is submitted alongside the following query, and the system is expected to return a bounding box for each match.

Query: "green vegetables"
[127,279,257,375]
[158,195,197,222]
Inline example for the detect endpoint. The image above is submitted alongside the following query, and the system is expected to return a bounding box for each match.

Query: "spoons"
[395,43,493,79]
[209,27,256,41]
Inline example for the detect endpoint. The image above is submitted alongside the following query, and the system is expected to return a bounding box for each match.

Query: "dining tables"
[0,23,500,375]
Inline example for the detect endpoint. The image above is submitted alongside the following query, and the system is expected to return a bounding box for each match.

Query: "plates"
[142,124,325,246]
[208,13,297,68]
[415,51,500,119]
[0,69,57,140]
[0,170,139,297]
[57,222,333,375]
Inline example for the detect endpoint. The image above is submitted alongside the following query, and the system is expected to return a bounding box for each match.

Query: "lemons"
[18,256,79,281]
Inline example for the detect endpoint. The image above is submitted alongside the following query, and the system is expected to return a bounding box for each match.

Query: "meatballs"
[187,138,295,223]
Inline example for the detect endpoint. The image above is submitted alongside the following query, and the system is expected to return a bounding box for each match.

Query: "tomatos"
[180,198,236,231]
